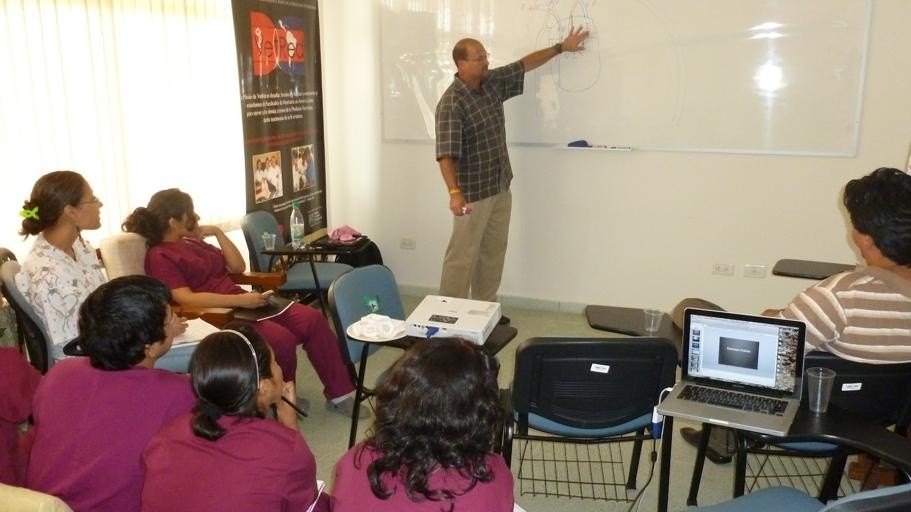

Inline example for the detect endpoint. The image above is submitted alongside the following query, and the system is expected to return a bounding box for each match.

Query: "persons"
[140,324,329,512]
[29,275,198,512]
[121,189,371,419]
[15,171,199,374]
[435,26,590,325]
[330,338,513,512]
[253,155,282,204]
[0,347,45,488]
[673,168,911,463]
[292,148,315,192]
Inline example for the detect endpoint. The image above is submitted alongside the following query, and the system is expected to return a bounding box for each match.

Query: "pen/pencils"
[179,307,184,317]
[280,395,309,417]
[261,290,274,296]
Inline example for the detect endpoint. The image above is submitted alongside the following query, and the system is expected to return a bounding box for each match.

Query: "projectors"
[405,293,503,347]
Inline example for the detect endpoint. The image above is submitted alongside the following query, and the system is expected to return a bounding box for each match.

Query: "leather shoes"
[681,427,732,463]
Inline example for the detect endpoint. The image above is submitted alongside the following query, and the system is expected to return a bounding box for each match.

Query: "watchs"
[555,44,561,53]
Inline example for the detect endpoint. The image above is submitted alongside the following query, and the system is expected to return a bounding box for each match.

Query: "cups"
[643,307,664,333]
[806,367,837,413]
[261,234,277,251]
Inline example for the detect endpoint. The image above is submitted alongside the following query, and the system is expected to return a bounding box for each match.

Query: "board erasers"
[568,140,587,147]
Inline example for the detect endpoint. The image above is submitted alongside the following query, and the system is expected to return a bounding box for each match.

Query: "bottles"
[289,202,306,250]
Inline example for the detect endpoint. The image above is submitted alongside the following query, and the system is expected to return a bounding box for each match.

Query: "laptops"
[656,306,807,439]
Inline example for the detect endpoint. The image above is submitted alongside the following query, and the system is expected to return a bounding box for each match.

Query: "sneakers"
[296,396,311,410]
[499,315,511,325]
[326,396,371,419]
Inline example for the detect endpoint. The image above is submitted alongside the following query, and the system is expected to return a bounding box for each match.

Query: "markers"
[587,145,607,148]
[462,208,472,211]
[463,211,470,214]
[610,147,630,149]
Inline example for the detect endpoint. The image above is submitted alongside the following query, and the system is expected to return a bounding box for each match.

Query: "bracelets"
[448,188,463,195]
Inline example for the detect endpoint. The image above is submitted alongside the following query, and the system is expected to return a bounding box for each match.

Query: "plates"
[346,318,408,343]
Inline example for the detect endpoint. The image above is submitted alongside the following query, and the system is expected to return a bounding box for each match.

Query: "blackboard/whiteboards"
[369,0,874,157]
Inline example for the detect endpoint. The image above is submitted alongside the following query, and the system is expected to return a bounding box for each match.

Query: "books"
[170,318,221,349]
[310,234,368,245]
[233,296,295,322]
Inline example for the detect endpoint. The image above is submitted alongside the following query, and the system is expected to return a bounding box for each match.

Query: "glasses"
[79,195,100,205]
[471,53,491,62]
